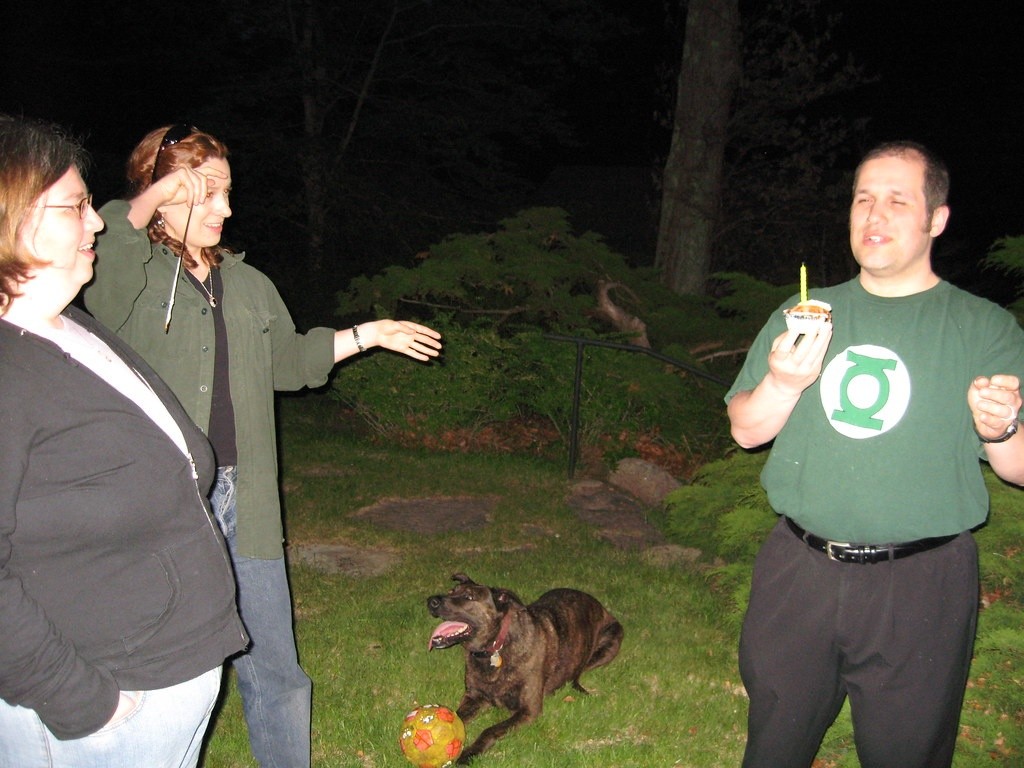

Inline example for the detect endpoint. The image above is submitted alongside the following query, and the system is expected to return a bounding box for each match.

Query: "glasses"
[151,122,201,185]
[24,194,93,220]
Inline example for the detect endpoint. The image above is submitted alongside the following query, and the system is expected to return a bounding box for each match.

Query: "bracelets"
[353,325,367,352]
[973,416,1019,444]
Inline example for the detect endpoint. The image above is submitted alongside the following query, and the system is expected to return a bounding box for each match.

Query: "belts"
[785,514,960,564]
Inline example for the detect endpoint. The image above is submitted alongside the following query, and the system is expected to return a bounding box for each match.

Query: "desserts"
[783,298,833,334]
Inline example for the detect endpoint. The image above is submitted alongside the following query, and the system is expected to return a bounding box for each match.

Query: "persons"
[0,116,249,768]
[723,142,1024,768]
[82,124,442,768]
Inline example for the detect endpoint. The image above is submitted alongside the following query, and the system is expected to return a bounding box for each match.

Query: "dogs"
[427,572,624,768]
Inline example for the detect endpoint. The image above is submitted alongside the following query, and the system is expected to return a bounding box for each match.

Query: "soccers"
[399,704,465,768]
